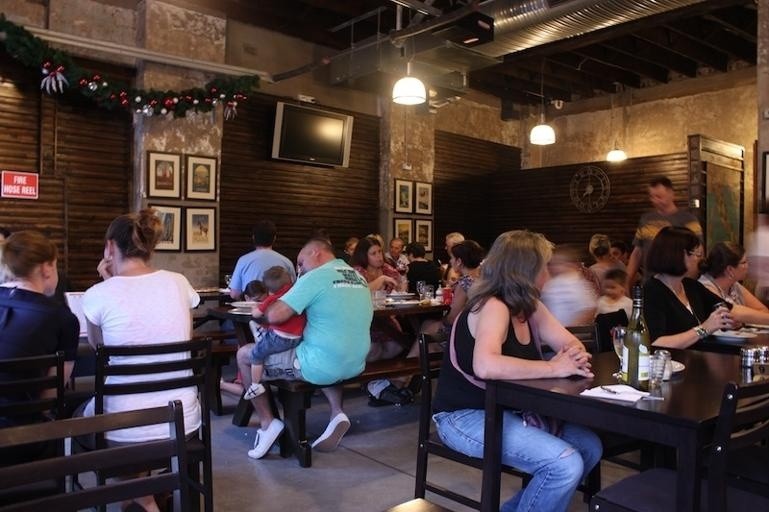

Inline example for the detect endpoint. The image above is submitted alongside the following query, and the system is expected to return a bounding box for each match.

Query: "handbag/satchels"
[450,290,565,438]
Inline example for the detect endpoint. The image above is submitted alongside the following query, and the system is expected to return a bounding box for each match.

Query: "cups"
[416,280,426,301]
[614,327,627,377]
[419,287,431,305]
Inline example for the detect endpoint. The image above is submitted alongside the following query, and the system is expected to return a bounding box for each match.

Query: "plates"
[385,301,420,307]
[230,302,256,307]
[227,309,252,316]
[671,359,687,372]
[385,293,416,300]
[712,331,758,342]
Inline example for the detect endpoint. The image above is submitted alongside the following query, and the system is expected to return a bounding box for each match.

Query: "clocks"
[569,166,611,214]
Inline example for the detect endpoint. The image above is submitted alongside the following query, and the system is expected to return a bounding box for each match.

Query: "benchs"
[210,343,242,415]
[275,356,449,468]
[194,329,238,346]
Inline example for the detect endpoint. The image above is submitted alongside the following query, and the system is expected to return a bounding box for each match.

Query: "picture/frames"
[393,218,413,246]
[414,181,433,216]
[147,151,182,199]
[147,203,182,252]
[394,178,414,214]
[184,153,218,202]
[414,218,433,253]
[184,207,217,252]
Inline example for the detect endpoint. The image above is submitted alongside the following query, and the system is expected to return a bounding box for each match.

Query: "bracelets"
[693,327,711,337]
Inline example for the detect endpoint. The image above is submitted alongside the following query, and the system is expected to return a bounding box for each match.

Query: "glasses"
[740,258,753,266]
[687,250,704,257]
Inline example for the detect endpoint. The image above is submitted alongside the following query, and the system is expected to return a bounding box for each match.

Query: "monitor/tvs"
[270,102,354,168]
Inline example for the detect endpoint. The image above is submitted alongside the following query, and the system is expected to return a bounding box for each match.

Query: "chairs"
[69,337,215,512]
[415,331,533,512]
[1,350,66,505]
[540,322,599,362]
[589,379,768,511]
[1,400,190,512]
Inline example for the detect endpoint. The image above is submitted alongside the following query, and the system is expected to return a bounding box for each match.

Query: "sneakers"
[313,412,350,450]
[244,383,266,400]
[247,418,284,457]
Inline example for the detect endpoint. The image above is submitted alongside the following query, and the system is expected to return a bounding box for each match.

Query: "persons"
[219,280,268,396]
[0,231,80,467]
[625,175,704,298]
[592,269,633,350]
[384,239,410,274]
[540,244,597,326]
[642,226,735,349]
[697,241,769,326]
[243,266,307,401]
[432,231,603,512]
[588,234,627,280]
[0,227,19,285]
[366,240,487,406]
[343,237,360,263]
[228,224,296,299]
[609,240,630,266]
[350,239,407,362]
[441,232,464,281]
[406,243,442,292]
[236,239,373,458]
[58,208,202,512]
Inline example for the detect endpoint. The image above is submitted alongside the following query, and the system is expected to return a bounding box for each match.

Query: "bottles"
[712,301,731,332]
[621,280,651,392]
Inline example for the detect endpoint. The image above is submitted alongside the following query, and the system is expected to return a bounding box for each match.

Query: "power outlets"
[297,94,306,102]
[307,95,316,104]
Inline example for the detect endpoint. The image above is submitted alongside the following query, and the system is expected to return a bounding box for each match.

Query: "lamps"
[528,62,558,146]
[392,59,426,106]
[404,162,413,171]
[607,140,627,162]
